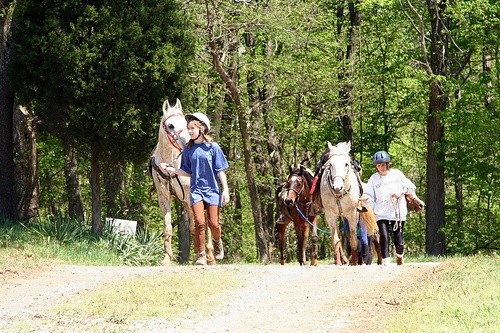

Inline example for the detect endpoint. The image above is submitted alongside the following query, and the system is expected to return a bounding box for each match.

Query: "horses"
[275,163,323,267]
[150,98,217,266]
[319,140,382,265]
[349,192,425,264]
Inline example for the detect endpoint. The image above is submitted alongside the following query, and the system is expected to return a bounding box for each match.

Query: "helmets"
[185,113,211,134]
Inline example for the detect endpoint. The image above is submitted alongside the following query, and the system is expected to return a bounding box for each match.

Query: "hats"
[373,151,391,164]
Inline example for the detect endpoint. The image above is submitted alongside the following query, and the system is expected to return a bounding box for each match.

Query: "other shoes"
[397,254,405,265]
[382,258,390,268]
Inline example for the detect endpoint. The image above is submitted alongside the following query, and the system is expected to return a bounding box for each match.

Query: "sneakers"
[212,240,225,259]
[197,252,208,264]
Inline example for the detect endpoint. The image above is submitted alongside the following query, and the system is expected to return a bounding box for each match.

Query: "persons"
[342,218,370,266]
[158,112,230,265]
[362,151,416,267]
